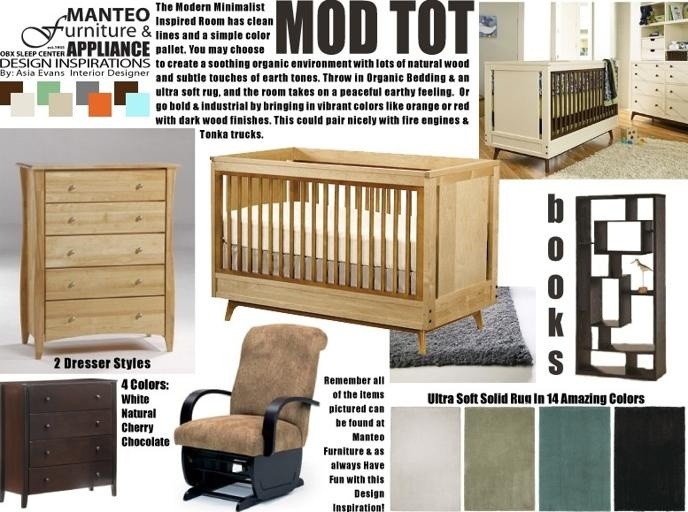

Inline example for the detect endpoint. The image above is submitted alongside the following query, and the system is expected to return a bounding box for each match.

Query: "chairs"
[172,324,331,512]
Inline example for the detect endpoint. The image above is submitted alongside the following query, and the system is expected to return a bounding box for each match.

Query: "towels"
[602,57,620,108]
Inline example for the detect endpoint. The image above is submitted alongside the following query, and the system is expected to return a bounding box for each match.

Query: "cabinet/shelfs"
[572,192,668,382]
[626,2,688,126]
[13,157,178,363]
[2,376,122,510]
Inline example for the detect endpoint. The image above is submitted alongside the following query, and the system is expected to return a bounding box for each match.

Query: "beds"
[482,58,621,175]
[206,143,499,360]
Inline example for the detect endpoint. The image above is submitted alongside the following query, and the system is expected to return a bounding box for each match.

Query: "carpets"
[549,134,687,179]
[388,283,533,369]
